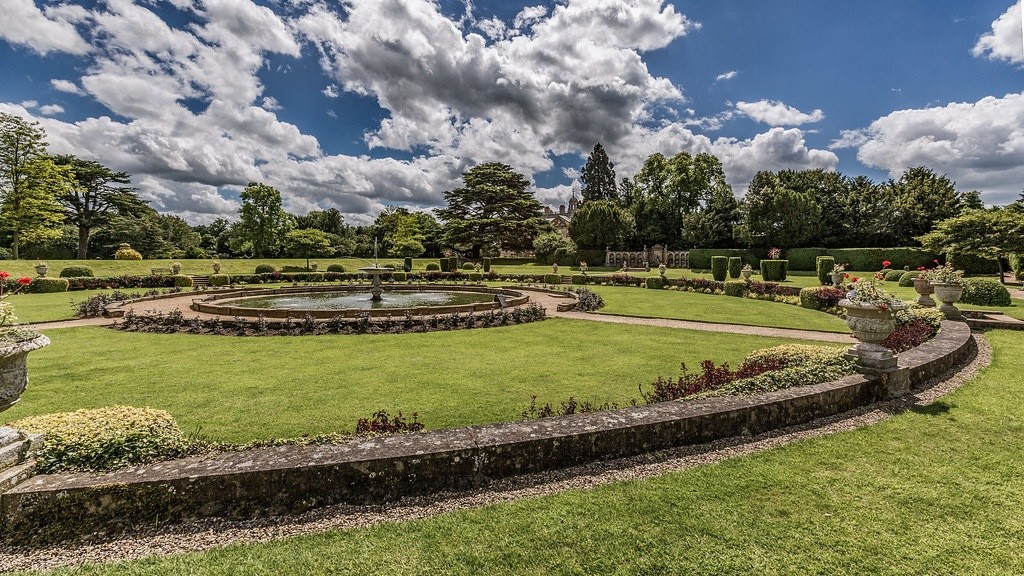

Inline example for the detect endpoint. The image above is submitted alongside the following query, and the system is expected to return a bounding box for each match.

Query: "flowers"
[768,247,781,257]
[0,271,42,346]
[844,260,897,313]
[743,263,752,270]
[832,263,849,273]
[917,259,964,284]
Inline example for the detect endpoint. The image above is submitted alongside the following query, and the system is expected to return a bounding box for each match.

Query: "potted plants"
[213,261,221,274]
[311,261,318,270]
[172,262,182,272]
[658,263,667,278]
[33,261,48,273]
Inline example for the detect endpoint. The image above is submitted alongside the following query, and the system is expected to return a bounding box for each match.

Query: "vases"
[0,334,51,413]
[742,270,753,278]
[911,278,964,302]
[828,273,846,283]
[837,298,902,343]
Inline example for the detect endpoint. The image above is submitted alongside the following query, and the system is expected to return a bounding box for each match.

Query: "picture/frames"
[772,255,780,260]
